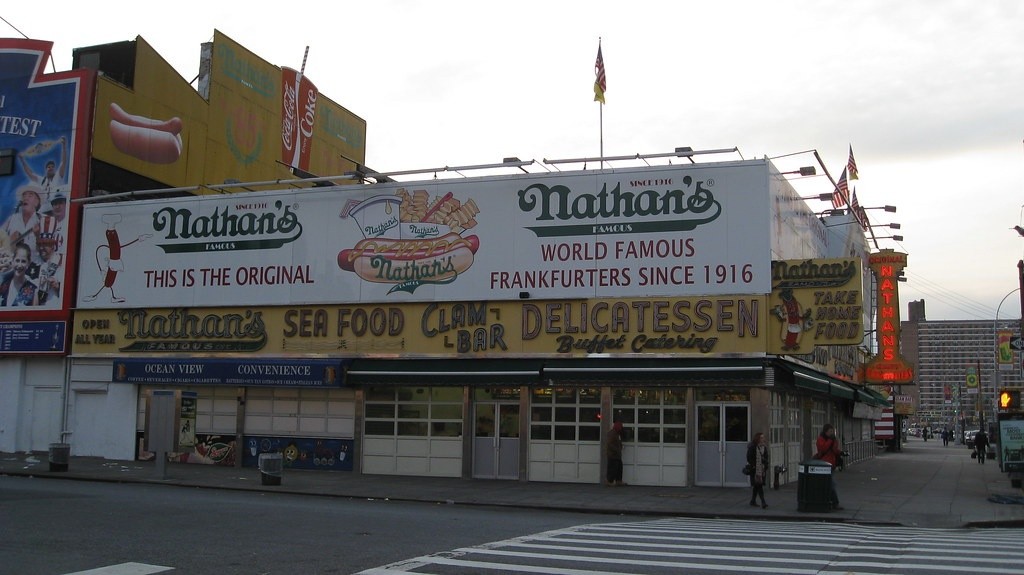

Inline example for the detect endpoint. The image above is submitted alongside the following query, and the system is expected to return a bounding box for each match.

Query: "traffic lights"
[998,390,1020,409]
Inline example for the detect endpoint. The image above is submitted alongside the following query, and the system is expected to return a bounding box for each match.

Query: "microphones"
[15,200,24,213]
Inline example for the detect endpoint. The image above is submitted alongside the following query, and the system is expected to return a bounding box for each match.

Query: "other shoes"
[750,500,760,506]
[762,502,768,508]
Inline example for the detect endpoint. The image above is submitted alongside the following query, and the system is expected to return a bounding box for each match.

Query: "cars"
[908,428,917,436]
[964,430,978,442]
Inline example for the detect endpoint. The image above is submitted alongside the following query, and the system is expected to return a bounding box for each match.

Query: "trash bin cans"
[48,443,71,471]
[797,459,834,512]
[258,453,283,487]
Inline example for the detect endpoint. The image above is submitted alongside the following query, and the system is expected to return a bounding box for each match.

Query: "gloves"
[841,450,849,456]
[829,434,834,440]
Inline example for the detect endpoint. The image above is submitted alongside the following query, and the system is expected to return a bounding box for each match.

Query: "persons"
[0,182,66,309]
[948,429,954,441]
[972,427,990,464]
[606,420,628,486]
[745,432,771,509]
[815,424,850,511]
[922,426,928,442]
[18,135,67,217]
[941,426,949,446]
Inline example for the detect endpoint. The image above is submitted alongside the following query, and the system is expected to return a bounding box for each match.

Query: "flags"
[852,193,860,212]
[858,208,869,231]
[594,45,607,104]
[831,168,848,209]
[847,146,858,180]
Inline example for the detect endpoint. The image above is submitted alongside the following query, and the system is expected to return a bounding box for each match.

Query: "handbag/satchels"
[812,453,820,460]
[743,463,754,475]
[971,448,976,458]
